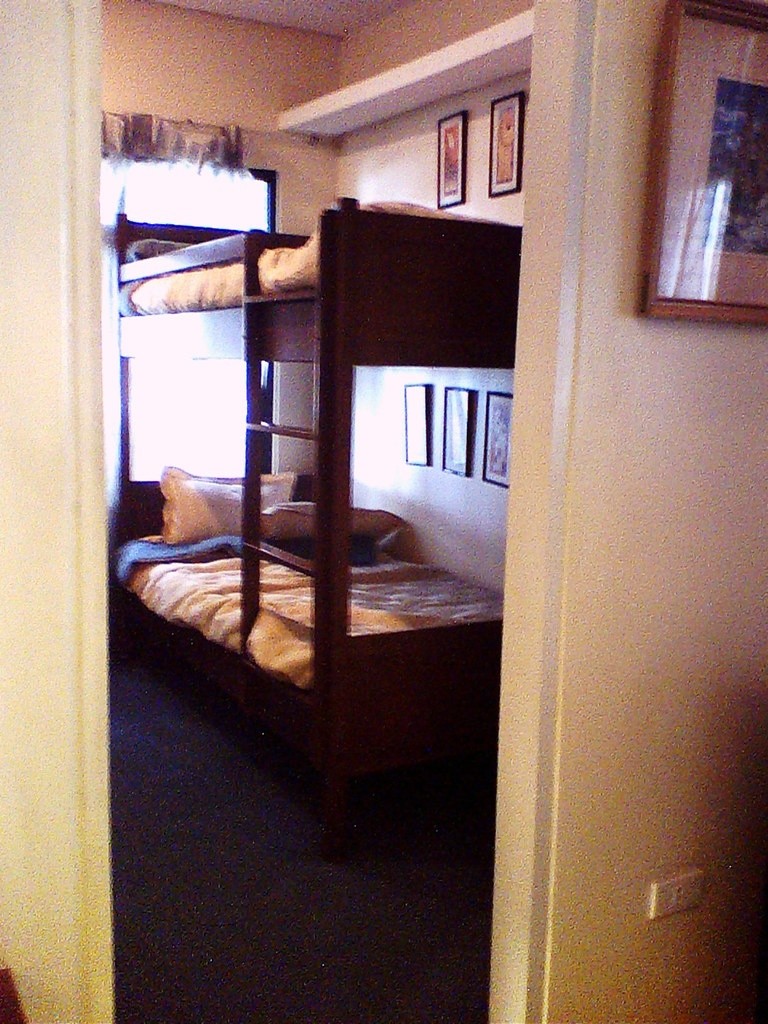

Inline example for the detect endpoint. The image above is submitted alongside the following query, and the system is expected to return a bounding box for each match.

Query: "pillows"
[126,239,196,264]
[158,467,297,547]
[251,499,407,558]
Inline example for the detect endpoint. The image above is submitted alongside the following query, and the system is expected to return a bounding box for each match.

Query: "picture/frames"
[639,1,768,324]
[482,390,513,489]
[436,110,468,209]
[488,90,526,199]
[441,387,476,478]
[404,383,432,468]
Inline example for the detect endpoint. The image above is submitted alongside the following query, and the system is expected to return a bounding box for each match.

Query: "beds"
[109,474,504,864]
[116,202,524,372]
